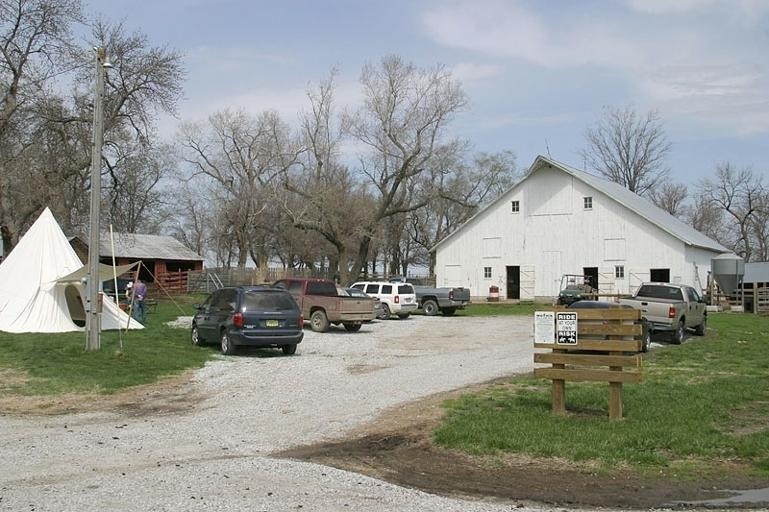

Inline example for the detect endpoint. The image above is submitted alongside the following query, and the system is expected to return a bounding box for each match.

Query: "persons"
[125,281,135,316]
[131,278,147,323]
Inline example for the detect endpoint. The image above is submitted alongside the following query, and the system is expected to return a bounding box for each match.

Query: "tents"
[0,205,148,335]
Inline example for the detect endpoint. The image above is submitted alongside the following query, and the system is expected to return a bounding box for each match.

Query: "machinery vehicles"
[558,274,598,304]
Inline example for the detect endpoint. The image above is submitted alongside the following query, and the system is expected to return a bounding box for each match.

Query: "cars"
[336,286,384,322]
[562,300,650,355]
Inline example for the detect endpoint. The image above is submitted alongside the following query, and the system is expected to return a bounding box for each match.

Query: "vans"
[350,281,420,320]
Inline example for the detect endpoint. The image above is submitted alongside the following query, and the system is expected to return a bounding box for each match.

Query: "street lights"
[86,49,115,350]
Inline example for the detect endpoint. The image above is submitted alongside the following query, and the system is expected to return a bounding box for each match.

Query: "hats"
[126,281,134,288]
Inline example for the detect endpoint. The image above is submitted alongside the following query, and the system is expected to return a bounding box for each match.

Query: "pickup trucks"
[269,277,376,332]
[618,282,709,344]
[388,278,471,315]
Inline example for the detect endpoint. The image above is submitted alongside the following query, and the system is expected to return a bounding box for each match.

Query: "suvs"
[192,284,303,356]
